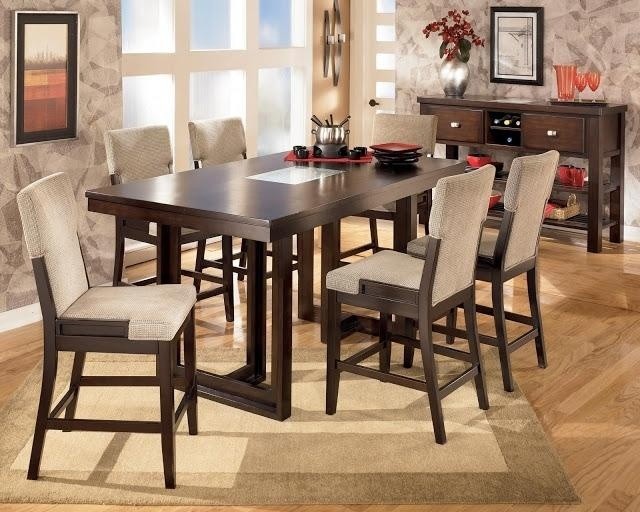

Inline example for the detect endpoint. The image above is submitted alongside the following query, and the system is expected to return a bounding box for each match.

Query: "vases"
[438,52,469,96]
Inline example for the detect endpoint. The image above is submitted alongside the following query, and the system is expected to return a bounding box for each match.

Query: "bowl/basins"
[489,191,501,210]
[467,154,491,167]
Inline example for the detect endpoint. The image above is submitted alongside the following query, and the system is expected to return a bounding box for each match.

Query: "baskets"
[544,193,583,220]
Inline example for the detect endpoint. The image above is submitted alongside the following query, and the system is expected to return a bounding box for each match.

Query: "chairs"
[402,141,561,395]
[326,158,497,439]
[183,114,300,276]
[16,171,201,488]
[98,124,235,326]
[338,113,439,266]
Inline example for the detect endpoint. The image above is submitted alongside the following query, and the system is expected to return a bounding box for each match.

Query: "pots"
[311,125,350,144]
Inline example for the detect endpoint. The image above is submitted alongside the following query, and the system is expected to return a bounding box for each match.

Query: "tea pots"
[552,64,577,102]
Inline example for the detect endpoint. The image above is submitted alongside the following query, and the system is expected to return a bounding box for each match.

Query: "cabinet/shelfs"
[416,93,629,255]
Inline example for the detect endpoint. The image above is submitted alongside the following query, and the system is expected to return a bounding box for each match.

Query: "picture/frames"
[490,5,545,86]
[10,12,80,147]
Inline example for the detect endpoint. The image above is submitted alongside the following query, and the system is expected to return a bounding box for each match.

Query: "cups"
[297,149,309,159]
[354,147,366,156]
[588,71,600,102]
[294,146,302,155]
[348,150,361,160]
[559,165,573,185]
[575,72,588,102]
[572,167,586,188]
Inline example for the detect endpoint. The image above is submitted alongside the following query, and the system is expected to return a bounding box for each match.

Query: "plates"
[369,142,422,153]
[283,149,372,164]
[551,98,609,106]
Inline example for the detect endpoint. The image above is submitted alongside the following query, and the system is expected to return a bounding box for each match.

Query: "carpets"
[0,336,578,505]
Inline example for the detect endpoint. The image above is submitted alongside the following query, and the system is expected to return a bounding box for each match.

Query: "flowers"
[418,7,486,63]
